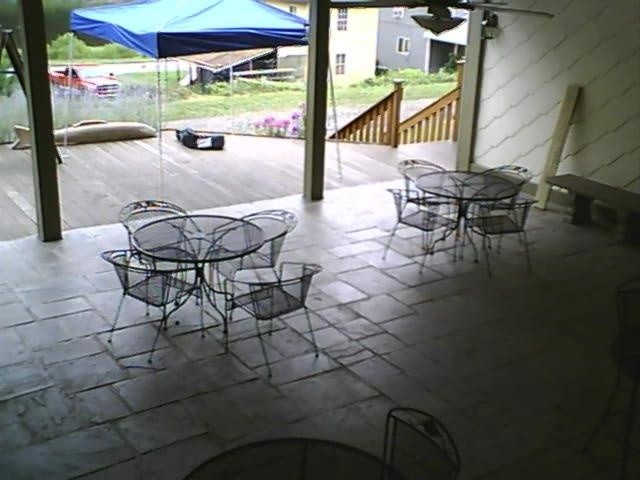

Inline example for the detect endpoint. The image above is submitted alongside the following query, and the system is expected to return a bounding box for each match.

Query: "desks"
[186,437,388,480]
[415,170,520,263]
[131,215,265,339]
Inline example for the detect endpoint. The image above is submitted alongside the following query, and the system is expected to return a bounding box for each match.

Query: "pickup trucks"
[50,64,123,105]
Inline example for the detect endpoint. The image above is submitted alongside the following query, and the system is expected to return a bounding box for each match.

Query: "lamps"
[412,7,467,35]
[481,14,498,39]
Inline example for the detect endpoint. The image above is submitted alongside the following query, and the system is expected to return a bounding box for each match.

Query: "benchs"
[542,173,640,244]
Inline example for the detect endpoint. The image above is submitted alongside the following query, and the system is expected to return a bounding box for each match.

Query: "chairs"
[383,406,462,480]
[457,164,534,255]
[101,249,205,364]
[382,188,461,275]
[397,159,462,250]
[222,262,322,380]
[460,199,539,278]
[210,209,298,323]
[118,199,204,332]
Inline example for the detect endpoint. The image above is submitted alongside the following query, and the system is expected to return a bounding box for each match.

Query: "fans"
[325,0,555,34]
